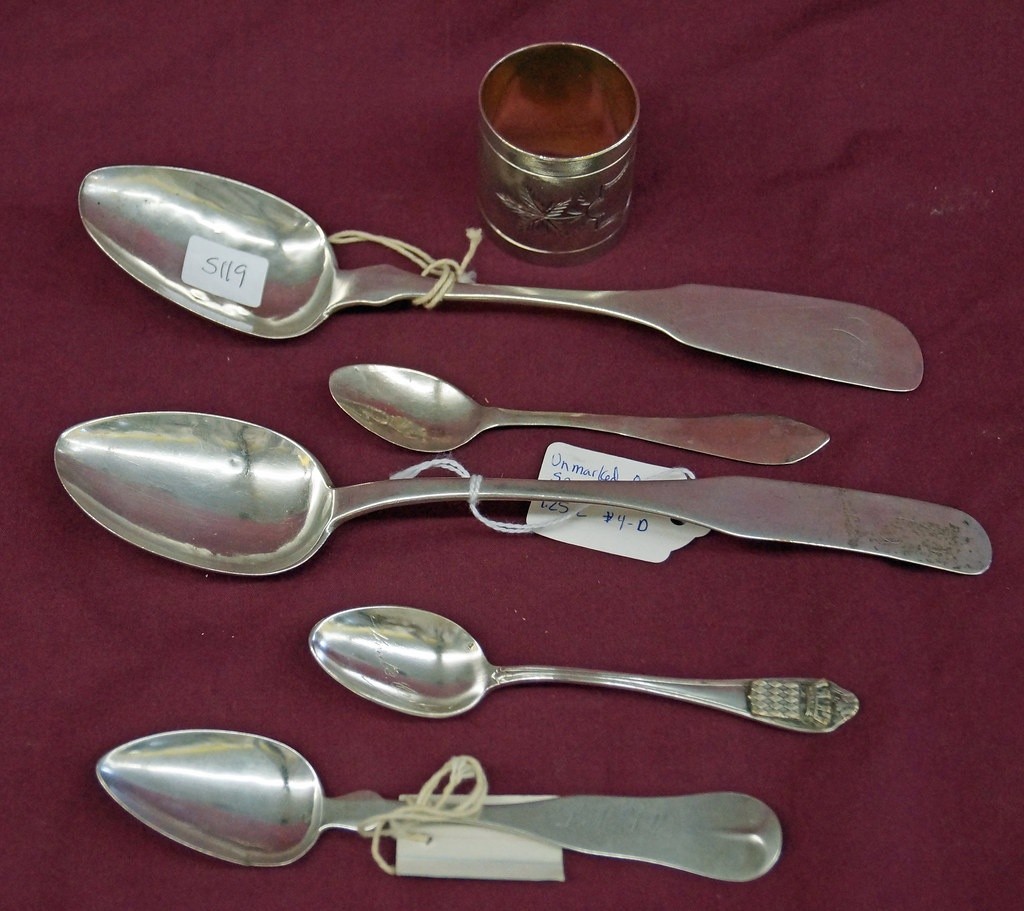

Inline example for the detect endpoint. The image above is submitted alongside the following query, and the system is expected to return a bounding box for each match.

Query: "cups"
[473,41,641,268]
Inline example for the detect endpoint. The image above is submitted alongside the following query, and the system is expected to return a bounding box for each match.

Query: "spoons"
[307,603,860,734]
[94,726,784,883]
[77,163,925,394]
[52,409,994,576]
[328,363,830,466]
